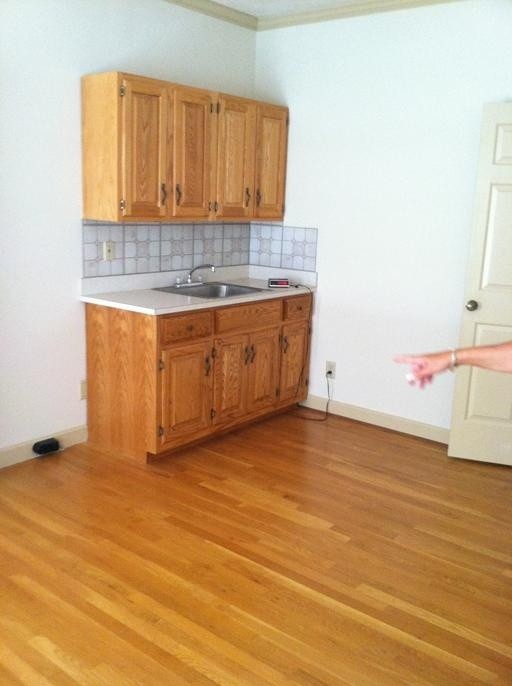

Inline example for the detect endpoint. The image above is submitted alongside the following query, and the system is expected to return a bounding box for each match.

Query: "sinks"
[145,281,275,299]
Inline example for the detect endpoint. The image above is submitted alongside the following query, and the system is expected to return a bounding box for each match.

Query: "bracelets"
[448,347,459,375]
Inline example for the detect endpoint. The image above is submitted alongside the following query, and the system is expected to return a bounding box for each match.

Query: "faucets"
[185,264,216,284]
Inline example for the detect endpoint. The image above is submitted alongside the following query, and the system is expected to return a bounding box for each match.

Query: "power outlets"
[325,360,337,381]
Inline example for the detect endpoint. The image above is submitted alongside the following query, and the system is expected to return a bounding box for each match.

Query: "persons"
[385,334,511,391]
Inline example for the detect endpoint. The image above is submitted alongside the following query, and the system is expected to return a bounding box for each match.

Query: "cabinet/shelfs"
[80,69,213,230]
[208,298,283,441]
[84,302,212,467]
[276,290,313,417]
[209,88,289,224]
